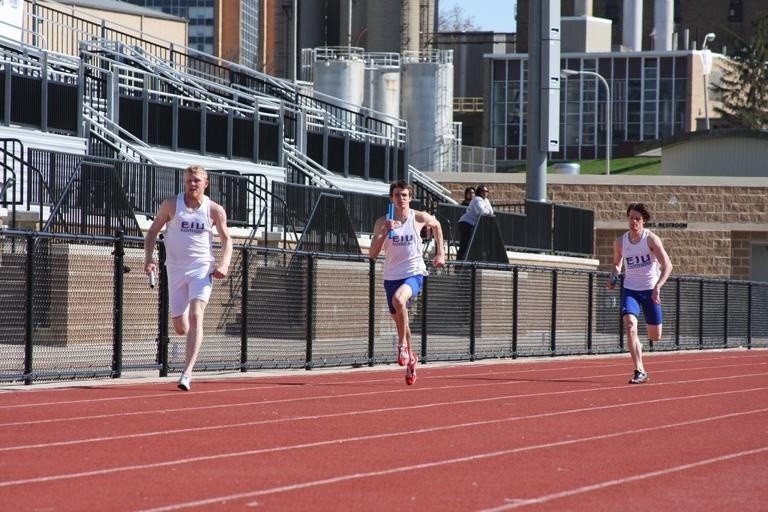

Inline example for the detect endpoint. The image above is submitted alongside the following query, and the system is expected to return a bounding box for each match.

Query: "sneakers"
[397,344,409,366]
[628,370,649,384]
[177,375,190,391]
[405,353,417,385]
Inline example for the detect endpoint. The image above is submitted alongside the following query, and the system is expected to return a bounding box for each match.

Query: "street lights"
[701,31,716,130]
[559,68,611,176]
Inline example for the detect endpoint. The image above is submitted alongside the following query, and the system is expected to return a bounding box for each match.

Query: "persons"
[144,164,234,391]
[368,178,445,385]
[454,185,494,274]
[459,186,476,206]
[608,202,674,384]
